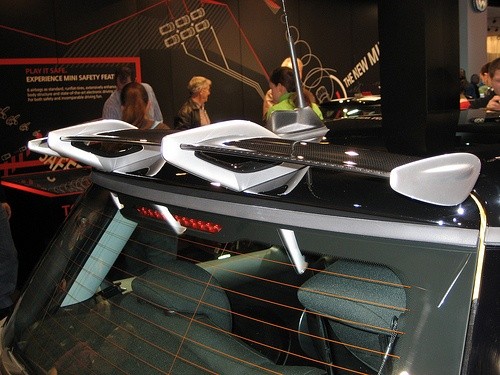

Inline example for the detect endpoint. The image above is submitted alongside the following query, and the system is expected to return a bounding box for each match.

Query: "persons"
[0,199,18,330]
[115,83,178,279]
[459,58,500,123]
[263,57,324,122]
[173,76,211,130]
[102,66,164,127]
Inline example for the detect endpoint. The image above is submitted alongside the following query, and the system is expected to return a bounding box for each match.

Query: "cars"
[317,97,381,121]
[1,118,500,373]
